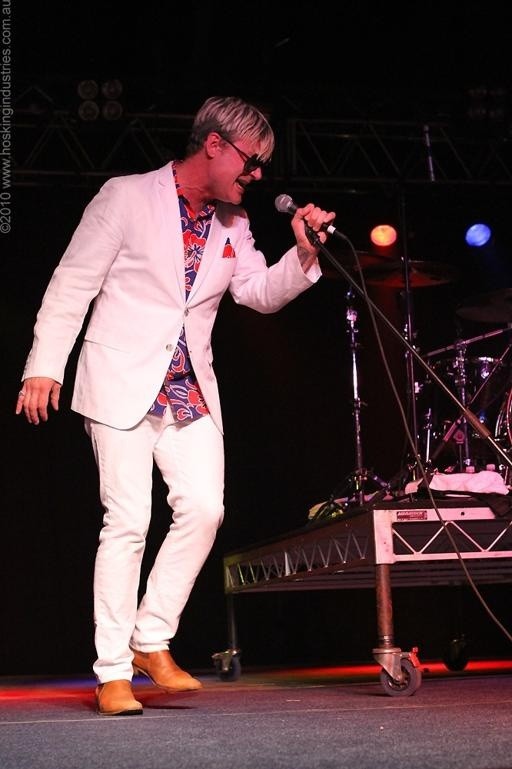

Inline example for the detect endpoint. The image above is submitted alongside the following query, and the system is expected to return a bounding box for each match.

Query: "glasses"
[217,133,270,172]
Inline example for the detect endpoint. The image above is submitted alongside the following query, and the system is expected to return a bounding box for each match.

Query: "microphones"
[274,192,337,238]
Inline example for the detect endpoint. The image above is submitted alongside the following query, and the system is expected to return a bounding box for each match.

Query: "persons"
[10,98,339,714]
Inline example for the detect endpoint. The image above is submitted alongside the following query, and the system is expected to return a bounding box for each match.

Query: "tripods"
[313,325,399,519]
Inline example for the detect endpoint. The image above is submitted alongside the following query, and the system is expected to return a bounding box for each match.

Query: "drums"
[494,391,512,469]
[415,357,506,439]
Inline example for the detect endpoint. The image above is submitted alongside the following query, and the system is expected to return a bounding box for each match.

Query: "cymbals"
[318,248,453,288]
[457,289,510,323]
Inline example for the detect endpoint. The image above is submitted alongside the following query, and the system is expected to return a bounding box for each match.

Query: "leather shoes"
[95,680,143,716]
[131,647,202,693]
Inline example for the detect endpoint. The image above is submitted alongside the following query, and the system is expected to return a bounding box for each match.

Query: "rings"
[18,391,25,396]
